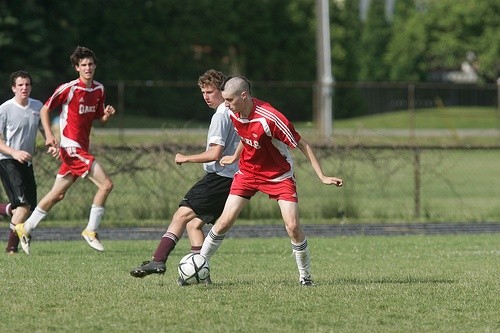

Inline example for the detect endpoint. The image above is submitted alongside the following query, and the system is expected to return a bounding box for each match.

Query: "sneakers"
[81,228,104,252]
[14,223,32,255]
[130,261,167,277]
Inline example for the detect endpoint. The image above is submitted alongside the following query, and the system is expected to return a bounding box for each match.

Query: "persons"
[177,76,343,285]
[0,71,60,253]
[15,46,115,254]
[130,71,241,283]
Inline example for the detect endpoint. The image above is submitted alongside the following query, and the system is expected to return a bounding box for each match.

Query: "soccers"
[178,253,210,284]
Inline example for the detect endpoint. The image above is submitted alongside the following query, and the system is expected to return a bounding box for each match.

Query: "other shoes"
[177,277,188,285]
[6,247,18,254]
[299,274,313,286]
